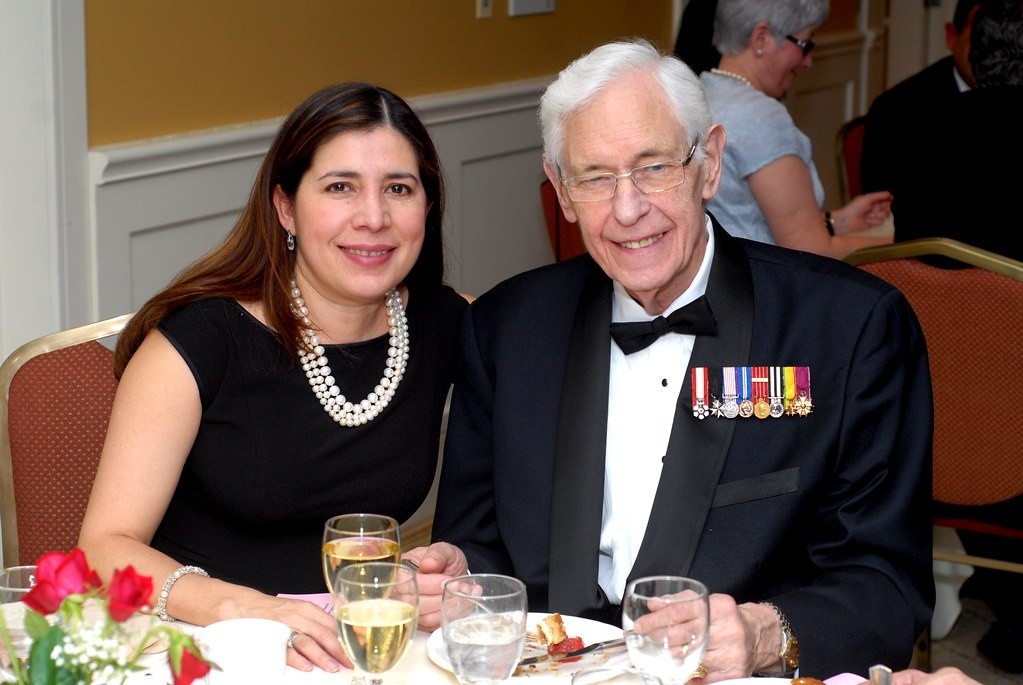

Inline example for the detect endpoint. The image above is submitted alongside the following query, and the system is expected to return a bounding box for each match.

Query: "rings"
[679,644,687,658]
[286,631,298,647]
[689,663,706,682]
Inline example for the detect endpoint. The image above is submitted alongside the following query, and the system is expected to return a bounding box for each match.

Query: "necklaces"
[710,67,753,88]
[285,276,408,426]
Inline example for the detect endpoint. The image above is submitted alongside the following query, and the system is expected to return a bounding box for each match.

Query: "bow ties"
[609,294,718,355]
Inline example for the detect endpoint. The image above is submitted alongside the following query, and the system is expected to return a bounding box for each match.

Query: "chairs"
[0,312,146,572]
[842,238,1023,676]
[541,111,864,265]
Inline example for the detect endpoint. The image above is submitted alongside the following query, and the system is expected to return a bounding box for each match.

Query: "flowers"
[0,549,223,685]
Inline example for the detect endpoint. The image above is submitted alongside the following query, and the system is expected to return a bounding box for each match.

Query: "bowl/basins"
[194,617,290,685]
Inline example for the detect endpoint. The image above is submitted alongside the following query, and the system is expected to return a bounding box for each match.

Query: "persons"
[865,0,1023,267]
[388,39,935,685]
[694,0,893,266]
[78,82,471,673]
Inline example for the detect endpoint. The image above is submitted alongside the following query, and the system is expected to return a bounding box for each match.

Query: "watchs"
[760,602,800,677]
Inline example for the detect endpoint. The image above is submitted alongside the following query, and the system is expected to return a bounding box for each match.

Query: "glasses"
[557,134,702,203]
[781,31,816,55]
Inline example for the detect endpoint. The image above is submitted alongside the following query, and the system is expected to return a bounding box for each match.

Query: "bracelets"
[824,210,835,236]
[157,565,210,622]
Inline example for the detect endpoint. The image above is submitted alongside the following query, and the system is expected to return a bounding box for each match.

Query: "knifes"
[518,637,626,667]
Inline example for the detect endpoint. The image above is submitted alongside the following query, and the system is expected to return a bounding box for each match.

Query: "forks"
[445,587,538,641]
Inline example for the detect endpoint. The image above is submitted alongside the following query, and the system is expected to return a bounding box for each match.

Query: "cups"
[442,574,528,685]
[622,574,710,685]
[0,566,56,658]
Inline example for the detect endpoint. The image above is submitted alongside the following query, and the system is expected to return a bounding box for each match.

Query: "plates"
[426,610,631,685]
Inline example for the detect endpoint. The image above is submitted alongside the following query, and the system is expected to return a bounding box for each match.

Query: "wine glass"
[322,513,401,685]
[331,562,418,685]
